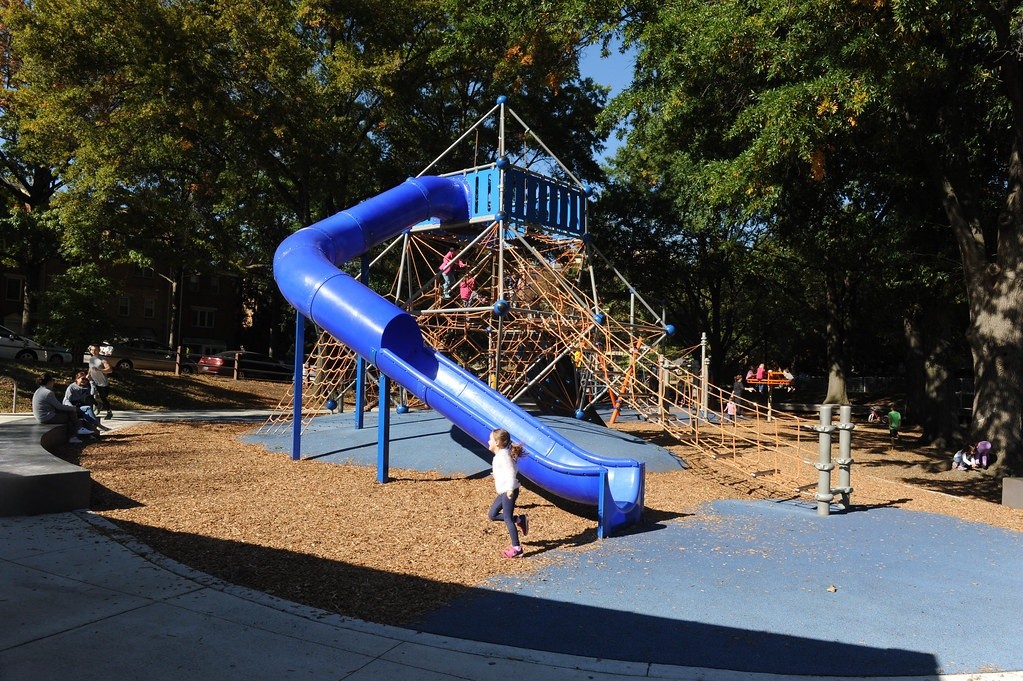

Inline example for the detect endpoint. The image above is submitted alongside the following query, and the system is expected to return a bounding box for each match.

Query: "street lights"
[211,258,273,346]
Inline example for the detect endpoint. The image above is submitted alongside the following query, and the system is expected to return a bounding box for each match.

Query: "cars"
[25,334,73,367]
[198,350,295,382]
[85,338,199,375]
[0,325,47,366]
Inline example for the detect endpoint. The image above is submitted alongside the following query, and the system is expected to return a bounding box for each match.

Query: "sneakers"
[78,426,94,435]
[68,436,82,443]
[502,547,523,558]
[518,513,529,537]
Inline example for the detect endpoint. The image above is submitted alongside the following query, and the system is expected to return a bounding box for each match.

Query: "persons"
[888,405,902,447]
[439,249,488,331]
[953,440,991,471]
[724,363,795,428]
[32,343,113,443]
[488,429,529,558]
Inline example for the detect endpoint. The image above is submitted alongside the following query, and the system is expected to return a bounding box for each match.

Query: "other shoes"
[105,412,114,420]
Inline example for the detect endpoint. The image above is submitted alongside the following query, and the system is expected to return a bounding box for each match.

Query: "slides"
[272,174,649,540]
